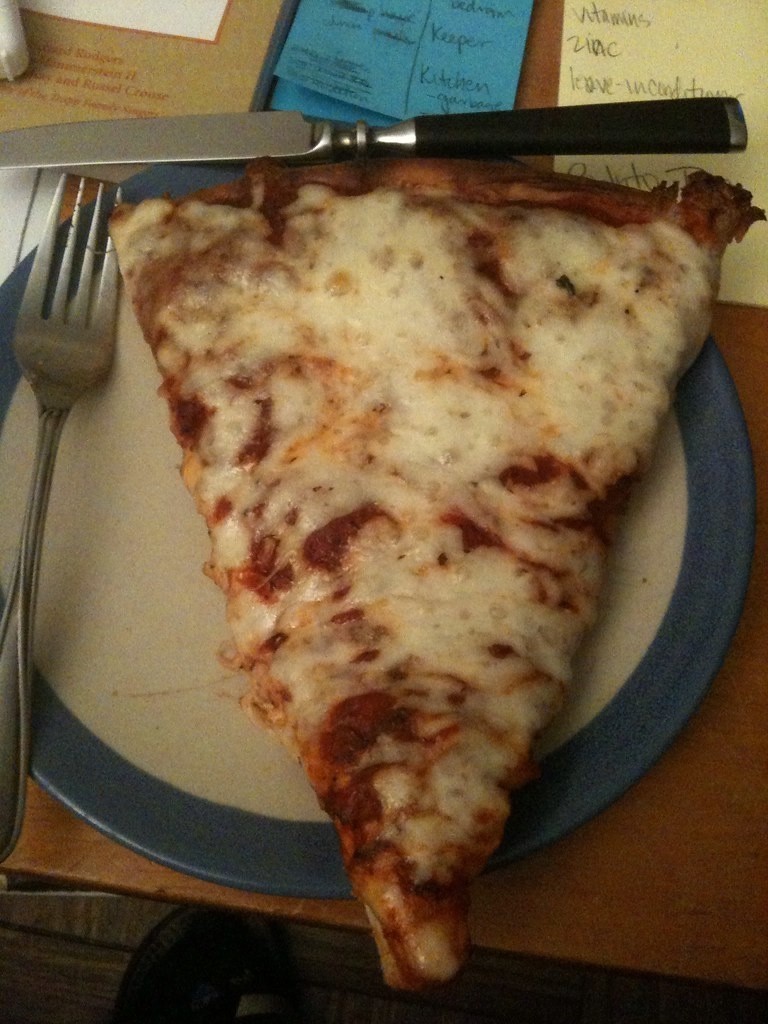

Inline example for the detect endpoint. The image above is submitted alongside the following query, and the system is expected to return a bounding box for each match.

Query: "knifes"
[2,96,746,195]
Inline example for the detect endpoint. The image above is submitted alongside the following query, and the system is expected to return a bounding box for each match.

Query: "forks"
[1,177,124,859]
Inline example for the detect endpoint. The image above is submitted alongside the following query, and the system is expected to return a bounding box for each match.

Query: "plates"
[0,196,753,897]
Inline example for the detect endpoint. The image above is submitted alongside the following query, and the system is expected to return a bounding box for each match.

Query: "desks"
[0,0,768,992]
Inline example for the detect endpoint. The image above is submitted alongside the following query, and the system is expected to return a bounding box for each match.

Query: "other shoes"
[120,905,300,1024]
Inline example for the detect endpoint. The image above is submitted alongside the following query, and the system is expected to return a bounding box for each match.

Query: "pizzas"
[105,157,768,993]
